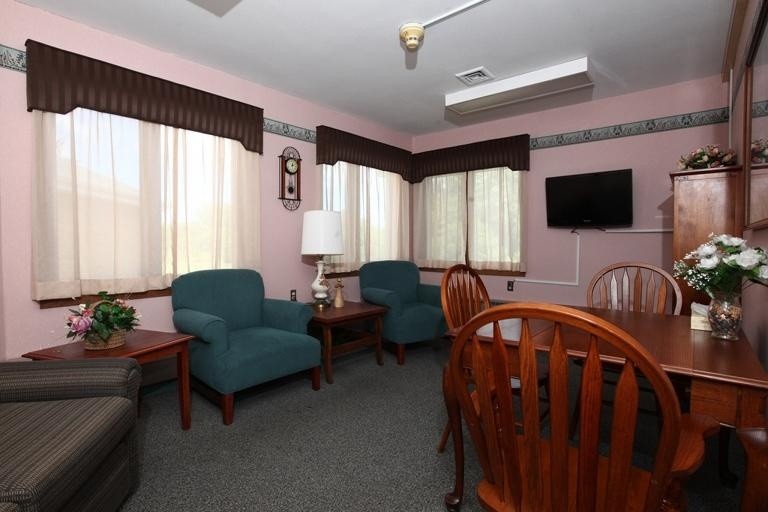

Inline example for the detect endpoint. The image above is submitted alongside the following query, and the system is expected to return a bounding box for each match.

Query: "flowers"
[674,144,736,169]
[751,137,768,164]
[674,232,768,336]
[63,290,142,344]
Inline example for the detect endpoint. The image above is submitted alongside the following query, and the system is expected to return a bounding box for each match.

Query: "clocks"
[285,158,298,175]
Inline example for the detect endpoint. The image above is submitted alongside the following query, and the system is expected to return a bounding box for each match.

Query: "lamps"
[301,210,344,308]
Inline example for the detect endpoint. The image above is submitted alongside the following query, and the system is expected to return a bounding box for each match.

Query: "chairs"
[359,261,449,366]
[586,261,682,319]
[450,303,682,511]
[172,269,321,425]
[437,264,549,454]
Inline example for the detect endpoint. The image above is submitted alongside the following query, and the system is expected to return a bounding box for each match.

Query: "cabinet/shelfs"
[750,164,768,225]
[670,165,744,316]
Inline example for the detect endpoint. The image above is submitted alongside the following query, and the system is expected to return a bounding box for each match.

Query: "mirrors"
[744,0,767,231]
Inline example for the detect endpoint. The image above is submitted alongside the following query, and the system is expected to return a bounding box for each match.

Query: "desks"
[446,305,768,512]
[307,300,387,384]
[22,329,194,430]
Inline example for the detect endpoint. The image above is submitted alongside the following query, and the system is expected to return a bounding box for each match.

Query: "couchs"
[0,359,142,512]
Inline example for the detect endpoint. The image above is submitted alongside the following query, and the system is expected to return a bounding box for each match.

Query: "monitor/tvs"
[545,167,633,230]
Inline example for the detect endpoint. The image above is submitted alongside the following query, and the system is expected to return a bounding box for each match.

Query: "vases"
[707,295,743,340]
